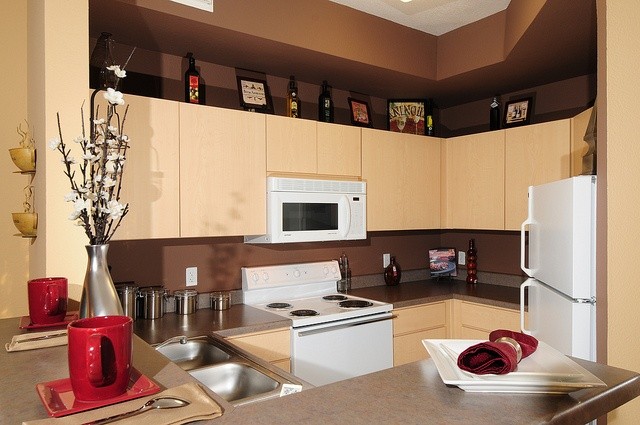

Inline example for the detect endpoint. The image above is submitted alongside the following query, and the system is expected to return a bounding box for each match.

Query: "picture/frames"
[347,95,375,129]
[236,74,272,111]
[387,97,427,137]
[500,95,534,127]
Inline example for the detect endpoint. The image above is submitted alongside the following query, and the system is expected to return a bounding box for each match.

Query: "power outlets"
[457,250,466,266]
[383,252,391,269]
[185,266,198,287]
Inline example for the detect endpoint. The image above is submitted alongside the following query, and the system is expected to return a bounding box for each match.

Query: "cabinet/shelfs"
[441,118,570,234]
[394,295,454,367]
[362,127,441,233]
[570,107,596,176]
[88,86,267,240]
[214,319,292,371]
[266,114,362,177]
[455,295,529,339]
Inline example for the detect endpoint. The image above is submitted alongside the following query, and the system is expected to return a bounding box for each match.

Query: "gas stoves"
[251,293,395,328]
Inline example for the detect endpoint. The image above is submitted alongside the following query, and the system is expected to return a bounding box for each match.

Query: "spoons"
[81,396,191,425]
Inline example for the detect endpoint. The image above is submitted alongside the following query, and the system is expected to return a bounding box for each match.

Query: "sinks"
[187,361,280,403]
[153,338,230,370]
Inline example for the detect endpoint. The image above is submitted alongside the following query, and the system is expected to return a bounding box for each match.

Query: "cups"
[67,316,133,401]
[27,277,68,325]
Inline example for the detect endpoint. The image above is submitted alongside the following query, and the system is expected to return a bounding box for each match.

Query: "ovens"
[289,312,393,388]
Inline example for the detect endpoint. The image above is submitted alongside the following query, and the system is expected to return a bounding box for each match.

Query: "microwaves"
[244,177,367,244]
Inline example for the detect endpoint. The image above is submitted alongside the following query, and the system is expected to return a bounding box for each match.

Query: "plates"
[36,366,160,417]
[18,314,78,329]
[421,338,608,395]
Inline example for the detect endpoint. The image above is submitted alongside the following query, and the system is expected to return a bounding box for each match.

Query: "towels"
[457,328,538,377]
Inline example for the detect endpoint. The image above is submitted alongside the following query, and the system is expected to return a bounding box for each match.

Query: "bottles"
[466,239,478,284]
[290,96,298,118]
[337,256,343,292]
[99,39,116,90]
[319,80,334,123]
[184,58,200,104]
[426,98,435,137]
[286,81,296,117]
[341,252,351,292]
[384,256,401,285]
[490,98,500,131]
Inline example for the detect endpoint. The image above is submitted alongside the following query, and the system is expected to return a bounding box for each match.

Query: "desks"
[1,308,640,425]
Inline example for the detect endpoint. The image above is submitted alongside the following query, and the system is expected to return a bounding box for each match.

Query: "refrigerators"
[519,175,597,425]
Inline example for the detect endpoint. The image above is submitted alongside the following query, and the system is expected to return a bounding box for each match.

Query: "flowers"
[49,46,136,242]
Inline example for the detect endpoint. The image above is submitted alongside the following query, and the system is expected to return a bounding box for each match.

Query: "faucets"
[155,335,187,351]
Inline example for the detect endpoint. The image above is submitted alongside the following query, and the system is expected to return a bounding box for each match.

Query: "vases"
[79,244,125,320]
